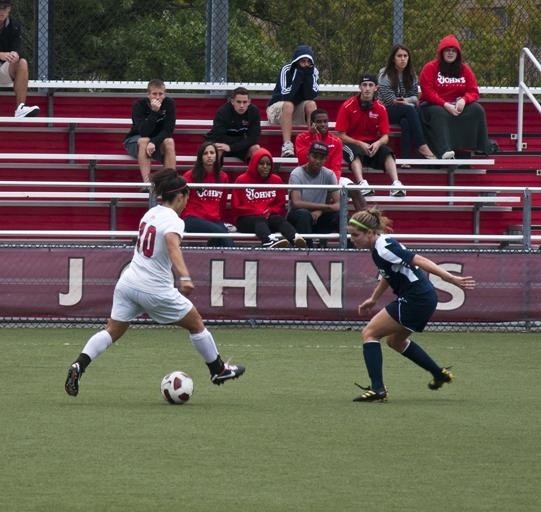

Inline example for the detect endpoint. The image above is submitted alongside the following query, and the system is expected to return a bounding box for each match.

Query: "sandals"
[418,148,436,159]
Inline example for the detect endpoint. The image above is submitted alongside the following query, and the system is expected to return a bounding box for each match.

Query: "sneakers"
[357,180,374,197]
[443,151,454,159]
[390,181,406,195]
[294,237,307,248]
[282,141,295,156]
[65,360,80,395]
[263,239,289,248]
[210,364,245,386]
[352,383,387,401]
[14,103,39,117]
[483,143,497,156]
[428,367,455,389]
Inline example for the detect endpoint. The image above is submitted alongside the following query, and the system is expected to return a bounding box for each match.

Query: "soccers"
[161,372,193,403]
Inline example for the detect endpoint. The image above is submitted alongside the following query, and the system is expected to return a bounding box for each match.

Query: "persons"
[418,35,496,164]
[65,167,245,397]
[0,1,41,120]
[177,144,238,246]
[294,108,344,184]
[124,79,179,191]
[289,143,355,249]
[202,87,261,163]
[345,208,476,401]
[265,43,320,158]
[336,75,406,196]
[375,44,438,171]
[232,149,307,247]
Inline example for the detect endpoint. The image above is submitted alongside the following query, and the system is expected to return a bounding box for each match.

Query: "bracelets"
[178,277,193,280]
